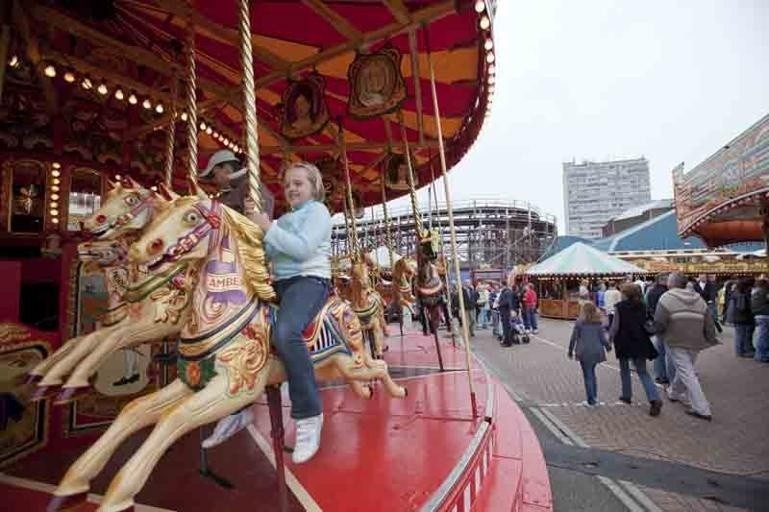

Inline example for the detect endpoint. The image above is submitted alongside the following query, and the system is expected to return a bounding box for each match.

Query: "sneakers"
[736,345,769,363]
[667,395,692,409]
[683,407,712,424]
[582,400,599,408]
[619,397,631,404]
[456,318,539,347]
[291,410,323,464]
[648,399,663,416]
[199,405,257,449]
[653,377,670,391]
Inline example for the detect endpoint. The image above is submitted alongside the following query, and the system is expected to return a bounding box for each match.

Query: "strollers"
[506,306,529,346]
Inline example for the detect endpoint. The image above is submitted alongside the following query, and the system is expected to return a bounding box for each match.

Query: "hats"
[197,149,241,177]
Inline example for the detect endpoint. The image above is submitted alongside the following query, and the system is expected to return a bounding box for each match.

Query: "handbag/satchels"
[703,305,716,346]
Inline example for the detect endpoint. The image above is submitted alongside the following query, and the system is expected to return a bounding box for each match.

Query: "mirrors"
[0,155,109,239]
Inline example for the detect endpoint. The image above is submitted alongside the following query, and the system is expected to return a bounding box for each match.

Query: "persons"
[607,283,665,418]
[442,278,540,349]
[647,271,724,421]
[196,159,334,464]
[567,300,612,406]
[573,270,769,364]
[198,148,276,222]
[645,272,675,384]
